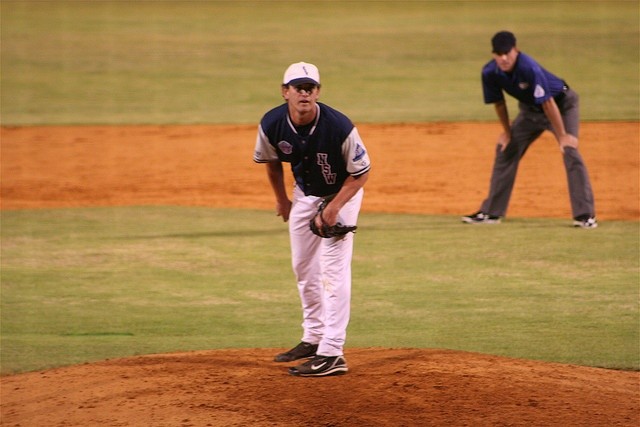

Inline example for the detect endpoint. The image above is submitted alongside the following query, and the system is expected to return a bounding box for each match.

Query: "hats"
[283,60,319,83]
[491,31,515,53]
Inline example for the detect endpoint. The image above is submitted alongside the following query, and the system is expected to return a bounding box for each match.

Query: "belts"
[555,76,570,98]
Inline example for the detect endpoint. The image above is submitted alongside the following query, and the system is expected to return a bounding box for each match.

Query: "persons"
[461,30,599,228]
[253,62,370,376]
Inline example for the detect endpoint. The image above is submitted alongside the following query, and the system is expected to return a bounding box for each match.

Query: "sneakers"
[287,354,351,377]
[462,212,504,224]
[571,216,597,227]
[276,341,320,361]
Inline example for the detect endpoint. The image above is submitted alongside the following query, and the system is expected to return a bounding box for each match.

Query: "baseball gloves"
[310,194,357,238]
